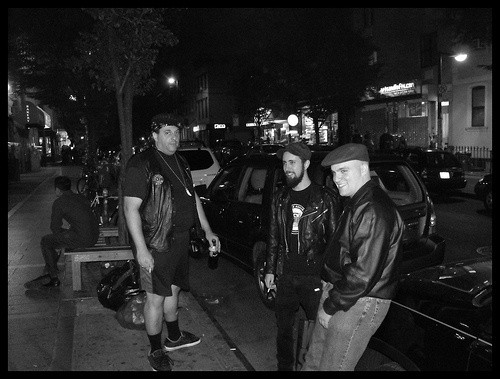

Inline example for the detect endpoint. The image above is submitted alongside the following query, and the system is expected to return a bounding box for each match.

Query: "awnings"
[24,101,52,129]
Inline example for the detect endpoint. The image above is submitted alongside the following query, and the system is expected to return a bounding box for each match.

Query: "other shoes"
[147,349,176,373]
[43,277,61,288]
[163,330,201,351]
[23,273,52,291]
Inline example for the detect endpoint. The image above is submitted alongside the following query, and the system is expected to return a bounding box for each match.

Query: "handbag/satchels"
[97,260,142,312]
[115,289,147,330]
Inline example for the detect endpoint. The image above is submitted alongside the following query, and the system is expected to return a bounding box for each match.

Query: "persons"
[121,116,221,371]
[261,142,337,371]
[25,175,100,288]
[302,142,401,371]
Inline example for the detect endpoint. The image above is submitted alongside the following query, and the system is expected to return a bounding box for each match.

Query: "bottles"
[211,239,218,257]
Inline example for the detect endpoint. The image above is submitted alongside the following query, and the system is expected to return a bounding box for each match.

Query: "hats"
[321,142,369,166]
[276,143,311,161]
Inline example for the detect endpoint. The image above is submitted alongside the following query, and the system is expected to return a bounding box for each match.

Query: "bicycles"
[77,155,102,205]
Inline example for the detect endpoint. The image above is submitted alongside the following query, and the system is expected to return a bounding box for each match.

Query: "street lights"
[435,49,469,151]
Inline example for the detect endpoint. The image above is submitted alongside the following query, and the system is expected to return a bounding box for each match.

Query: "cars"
[392,255,492,370]
[474,169,493,210]
[399,148,469,195]
[245,143,286,158]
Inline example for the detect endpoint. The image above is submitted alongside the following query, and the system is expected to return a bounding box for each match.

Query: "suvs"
[174,139,221,195]
[187,151,447,310]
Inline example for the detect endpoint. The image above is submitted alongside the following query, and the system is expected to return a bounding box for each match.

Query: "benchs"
[64,227,137,291]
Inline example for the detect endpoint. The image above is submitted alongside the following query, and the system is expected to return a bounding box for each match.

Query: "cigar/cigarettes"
[267,285,271,293]
